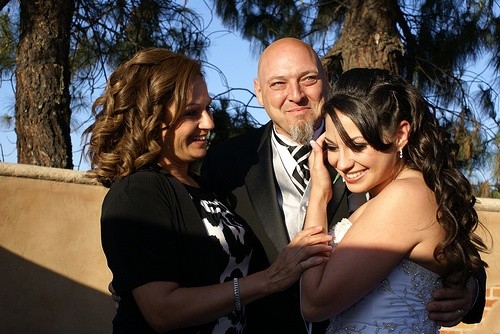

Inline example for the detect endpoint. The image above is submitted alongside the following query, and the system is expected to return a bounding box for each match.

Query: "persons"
[80,46,335,334]
[299,67,495,333]
[107,36,486,334]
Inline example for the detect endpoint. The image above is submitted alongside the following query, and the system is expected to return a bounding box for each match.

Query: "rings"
[457,308,462,318]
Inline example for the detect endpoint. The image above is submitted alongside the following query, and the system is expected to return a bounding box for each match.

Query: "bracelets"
[232,277,242,312]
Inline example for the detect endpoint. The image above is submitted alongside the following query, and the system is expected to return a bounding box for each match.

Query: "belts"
[271,116,325,196]
